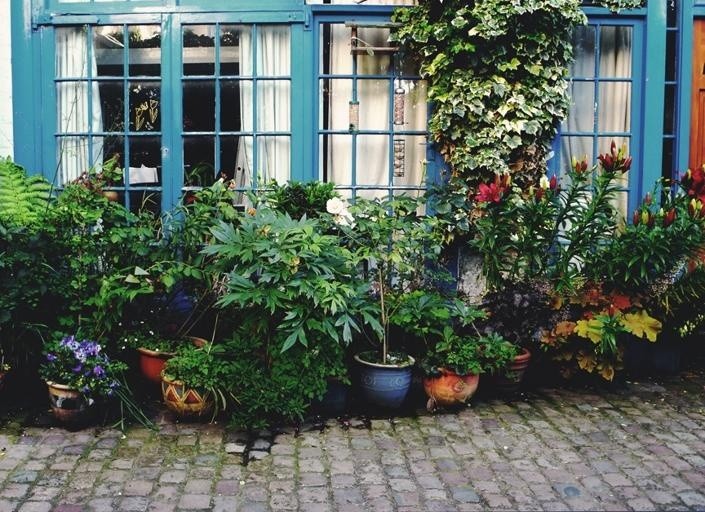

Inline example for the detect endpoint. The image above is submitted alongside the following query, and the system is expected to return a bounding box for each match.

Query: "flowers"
[38,333,123,406]
[486,151,663,378]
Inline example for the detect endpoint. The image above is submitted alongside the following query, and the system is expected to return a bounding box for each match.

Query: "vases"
[46,377,98,430]
[489,340,532,394]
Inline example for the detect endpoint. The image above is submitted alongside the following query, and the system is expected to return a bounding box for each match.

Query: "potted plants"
[613,202,663,382]
[649,185,694,376]
[161,345,225,423]
[119,195,219,386]
[348,199,417,416]
[417,292,484,418]
